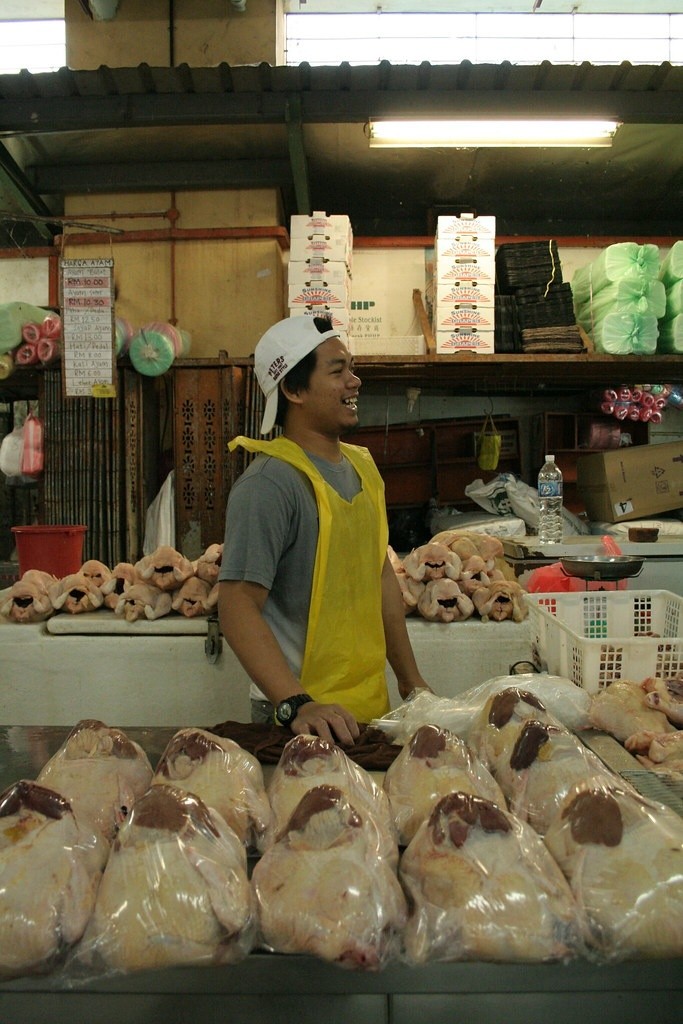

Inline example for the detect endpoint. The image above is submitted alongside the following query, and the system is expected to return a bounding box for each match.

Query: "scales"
[559,554,647,638]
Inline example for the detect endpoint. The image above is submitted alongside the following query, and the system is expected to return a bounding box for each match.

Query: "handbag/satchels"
[473,414,502,470]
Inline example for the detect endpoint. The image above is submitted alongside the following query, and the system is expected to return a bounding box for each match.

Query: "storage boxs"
[432,258,495,290]
[577,441,683,523]
[432,281,494,315]
[290,235,354,271]
[434,213,496,240]
[430,329,496,354]
[290,211,354,249]
[288,280,351,309]
[523,589,683,696]
[431,303,495,336]
[430,512,526,541]
[337,331,350,350]
[290,305,352,331]
[431,236,495,263]
[288,258,352,289]
[349,335,426,355]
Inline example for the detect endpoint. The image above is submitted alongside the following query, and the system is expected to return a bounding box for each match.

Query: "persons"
[218,312,437,746]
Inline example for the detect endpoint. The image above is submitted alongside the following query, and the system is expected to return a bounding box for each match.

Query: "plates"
[559,555,646,580]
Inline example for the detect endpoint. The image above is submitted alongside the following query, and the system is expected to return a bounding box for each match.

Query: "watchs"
[275,693,315,728]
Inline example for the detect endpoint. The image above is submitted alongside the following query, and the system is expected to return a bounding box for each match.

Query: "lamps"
[364,116,624,149]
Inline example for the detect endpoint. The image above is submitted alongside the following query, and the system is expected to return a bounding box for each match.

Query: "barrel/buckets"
[11,524,89,580]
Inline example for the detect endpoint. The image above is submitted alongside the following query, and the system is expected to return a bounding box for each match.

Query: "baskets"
[523,589,683,728]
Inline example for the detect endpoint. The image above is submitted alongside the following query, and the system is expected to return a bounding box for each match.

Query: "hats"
[254,315,340,434]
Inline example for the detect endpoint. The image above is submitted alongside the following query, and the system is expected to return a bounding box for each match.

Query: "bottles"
[536,454,564,544]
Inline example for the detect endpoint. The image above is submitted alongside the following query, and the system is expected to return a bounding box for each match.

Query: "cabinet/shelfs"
[532,411,647,514]
[340,416,524,555]
